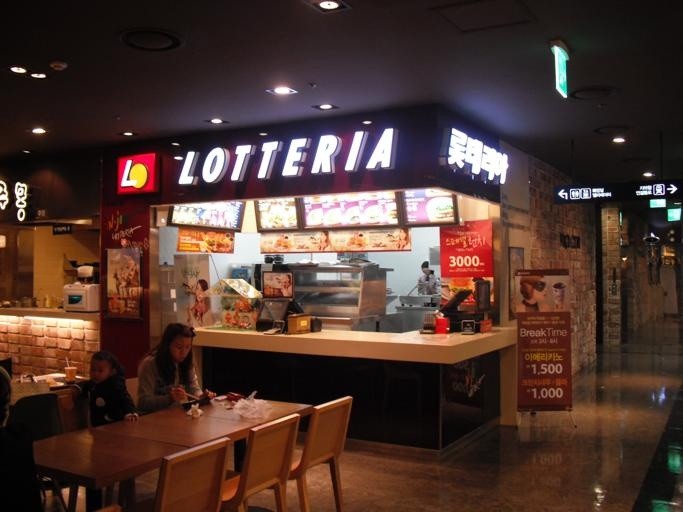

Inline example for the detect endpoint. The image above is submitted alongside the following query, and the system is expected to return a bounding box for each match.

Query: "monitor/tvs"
[286,298,304,313]
[439,290,472,321]
[256,299,289,331]
[477,281,490,312]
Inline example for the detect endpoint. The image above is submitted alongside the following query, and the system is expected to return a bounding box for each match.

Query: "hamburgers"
[276,240,288,249]
[348,237,364,249]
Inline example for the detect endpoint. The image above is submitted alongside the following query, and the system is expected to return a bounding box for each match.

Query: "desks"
[26,428,190,509]
[97,395,313,474]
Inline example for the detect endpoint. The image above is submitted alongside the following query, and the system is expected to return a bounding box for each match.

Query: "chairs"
[3,356,141,432]
[220,413,301,510]
[121,436,231,510]
[288,395,353,510]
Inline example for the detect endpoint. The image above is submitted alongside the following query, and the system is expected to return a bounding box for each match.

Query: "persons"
[135,322,218,414]
[416,261,438,308]
[518,274,551,314]
[395,227,410,250]
[281,274,292,290]
[186,278,209,324]
[114,255,140,295]
[316,231,329,250]
[68,350,139,429]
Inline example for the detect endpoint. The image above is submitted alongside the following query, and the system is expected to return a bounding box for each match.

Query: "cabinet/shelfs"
[272,263,394,331]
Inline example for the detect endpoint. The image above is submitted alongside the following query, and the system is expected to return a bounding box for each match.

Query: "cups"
[64,366,77,384]
[552,283,567,309]
